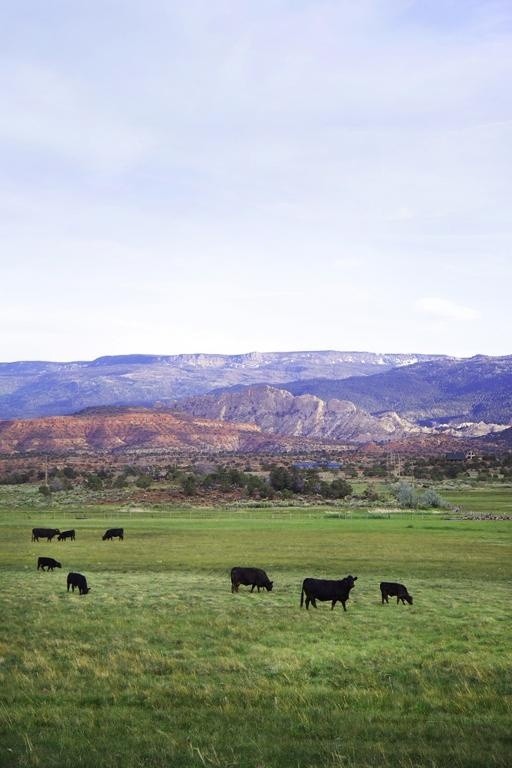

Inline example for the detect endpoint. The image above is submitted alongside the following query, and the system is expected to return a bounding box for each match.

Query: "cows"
[36,556,62,572]
[57,529,77,541]
[65,572,91,595]
[31,527,62,542]
[230,567,274,593]
[379,581,414,605]
[298,574,358,613]
[102,527,124,542]
